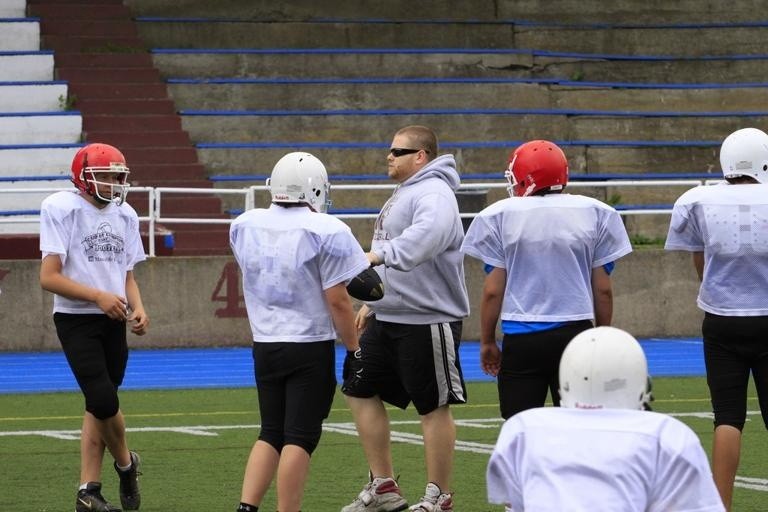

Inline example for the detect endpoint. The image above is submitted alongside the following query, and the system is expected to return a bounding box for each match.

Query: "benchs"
[0,0,176,254]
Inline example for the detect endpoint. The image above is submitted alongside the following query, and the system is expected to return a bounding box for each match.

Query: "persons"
[484,324,726,512]
[38,143,152,512]
[341,123,472,512]
[459,137,636,422]
[228,151,371,512]
[661,127,767,512]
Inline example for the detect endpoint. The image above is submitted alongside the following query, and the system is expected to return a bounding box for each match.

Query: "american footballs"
[347,267,384,300]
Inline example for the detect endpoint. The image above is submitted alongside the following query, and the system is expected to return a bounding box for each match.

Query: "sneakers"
[406,483,452,511]
[341,469,408,511]
[74,481,120,511]
[112,452,142,511]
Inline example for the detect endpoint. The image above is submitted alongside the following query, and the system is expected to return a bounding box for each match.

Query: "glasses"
[389,146,429,156]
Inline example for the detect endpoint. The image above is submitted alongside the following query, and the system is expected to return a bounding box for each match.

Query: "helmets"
[267,150,331,213]
[504,140,570,196]
[72,142,129,207]
[559,326,652,409]
[718,126,768,180]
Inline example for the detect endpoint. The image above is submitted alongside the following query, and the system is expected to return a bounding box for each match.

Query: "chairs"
[123,0,768,253]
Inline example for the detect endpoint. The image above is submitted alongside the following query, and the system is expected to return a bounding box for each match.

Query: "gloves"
[341,359,367,394]
